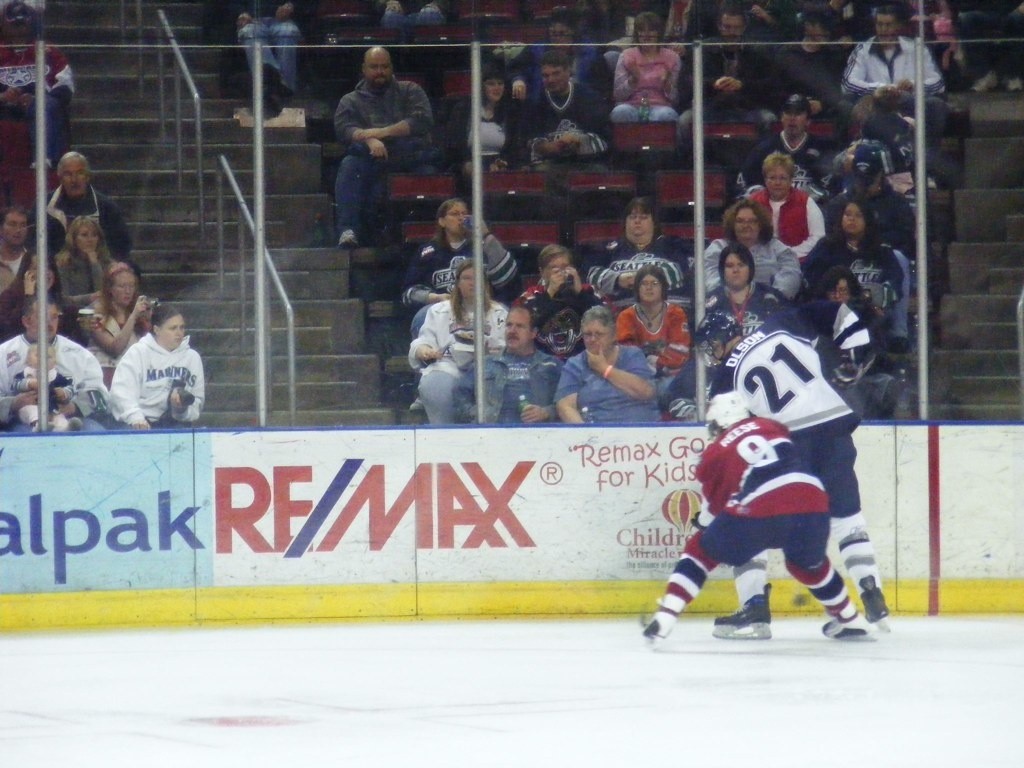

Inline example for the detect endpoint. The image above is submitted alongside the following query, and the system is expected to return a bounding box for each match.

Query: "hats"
[852,143,884,180]
[784,93,810,114]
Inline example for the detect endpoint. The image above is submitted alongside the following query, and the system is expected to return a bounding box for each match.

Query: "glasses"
[640,281,662,286]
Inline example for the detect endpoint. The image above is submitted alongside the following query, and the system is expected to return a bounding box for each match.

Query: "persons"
[639,392,875,646]
[2,3,209,432]
[697,302,891,624]
[232,1,1019,423]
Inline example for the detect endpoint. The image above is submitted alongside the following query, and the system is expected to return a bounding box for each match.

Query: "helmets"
[706,391,750,438]
[0,1,37,35]
[688,306,742,368]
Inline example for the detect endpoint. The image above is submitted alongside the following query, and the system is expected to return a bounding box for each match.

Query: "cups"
[78,309,95,336]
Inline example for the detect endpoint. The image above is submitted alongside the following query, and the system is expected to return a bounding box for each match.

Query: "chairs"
[565,169,639,195]
[609,117,675,155]
[686,119,759,144]
[401,222,436,242]
[572,220,624,246]
[0,117,61,211]
[313,0,652,100]
[489,221,560,250]
[386,171,457,219]
[483,171,549,197]
[654,165,732,212]
[769,118,837,138]
[659,221,727,239]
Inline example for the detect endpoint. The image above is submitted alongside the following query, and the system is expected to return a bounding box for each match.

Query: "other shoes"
[409,397,424,412]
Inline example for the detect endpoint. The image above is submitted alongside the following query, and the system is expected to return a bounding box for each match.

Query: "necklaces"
[639,305,663,328]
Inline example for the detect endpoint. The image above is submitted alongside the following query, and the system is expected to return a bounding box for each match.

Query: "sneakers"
[644,607,680,651]
[712,583,773,640]
[822,598,879,642]
[858,575,892,634]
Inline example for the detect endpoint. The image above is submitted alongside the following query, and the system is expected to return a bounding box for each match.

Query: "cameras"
[142,297,160,308]
[561,270,573,286]
[167,379,195,406]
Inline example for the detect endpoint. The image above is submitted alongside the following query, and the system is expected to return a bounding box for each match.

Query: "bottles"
[580,407,597,423]
[894,369,910,419]
[638,92,651,123]
[911,315,919,352]
[518,394,530,415]
[909,260,917,296]
[463,217,473,230]
[326,34,337,45]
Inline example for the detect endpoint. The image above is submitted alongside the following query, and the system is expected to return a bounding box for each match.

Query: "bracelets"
[604,365,613,378]
